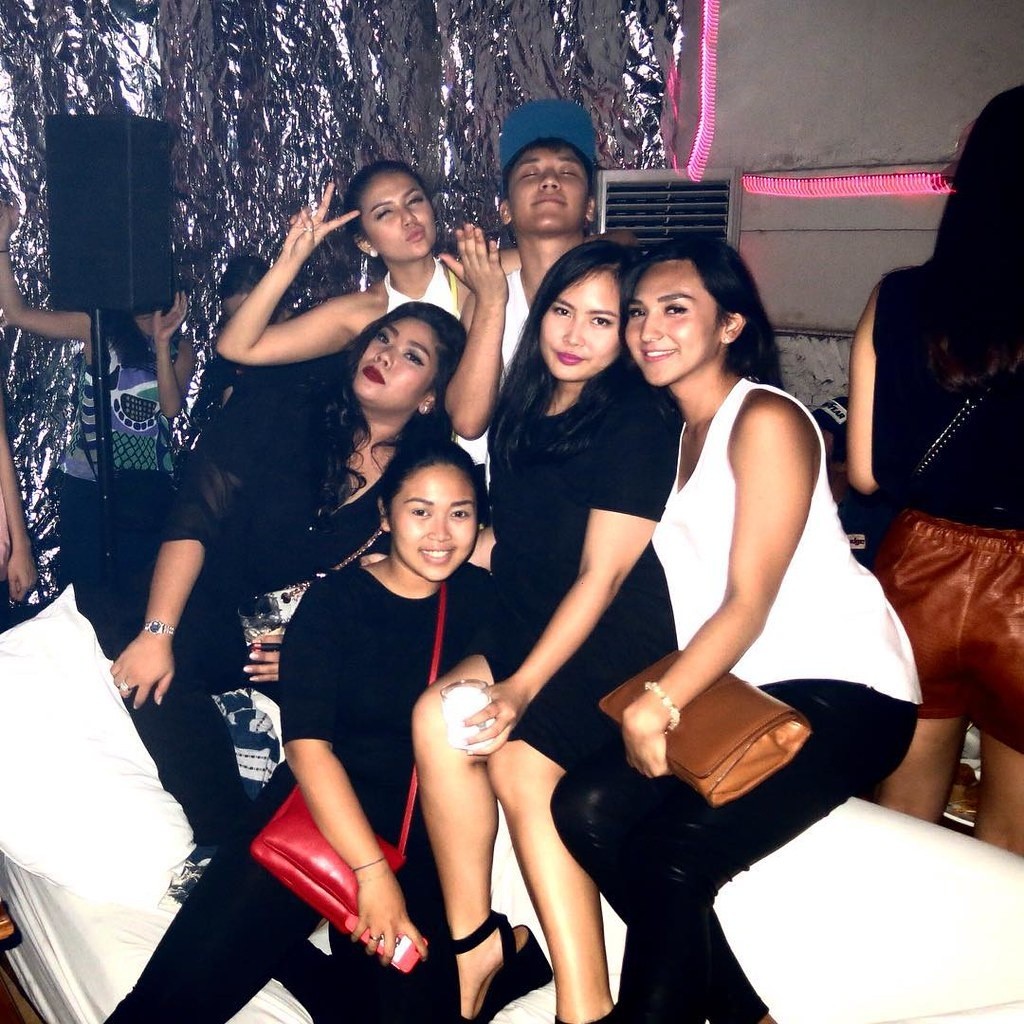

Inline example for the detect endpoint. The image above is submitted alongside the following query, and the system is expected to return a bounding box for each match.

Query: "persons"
[847,85,1024,856]
[437,135,597,471]
[102,441,492,1024]
[411,235,681,1024]
[0,389,37,633]
[216,158,638,366]
[110,302,491,900]
[0,197,195,661]
[550,233,920,1024]
[220,256,296,412]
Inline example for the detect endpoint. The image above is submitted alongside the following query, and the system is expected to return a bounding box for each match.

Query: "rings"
[120,680,134,691]
[369,935,382,941]
[304,226,314,232]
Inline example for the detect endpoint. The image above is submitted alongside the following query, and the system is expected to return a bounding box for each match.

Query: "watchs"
[143,619,176,636]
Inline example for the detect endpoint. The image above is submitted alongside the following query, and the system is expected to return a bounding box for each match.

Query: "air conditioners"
[594,168,744,259]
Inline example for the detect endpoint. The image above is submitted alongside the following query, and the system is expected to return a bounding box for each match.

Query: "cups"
[237,596,282,646]
[440,678,497,750]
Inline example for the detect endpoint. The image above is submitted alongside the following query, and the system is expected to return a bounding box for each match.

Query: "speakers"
[45,113,177,315]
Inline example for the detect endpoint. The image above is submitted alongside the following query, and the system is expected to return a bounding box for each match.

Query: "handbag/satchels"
[235,580,315,659]
[599,650,811,806]
[249,782,406,934]
[838,483,905,568]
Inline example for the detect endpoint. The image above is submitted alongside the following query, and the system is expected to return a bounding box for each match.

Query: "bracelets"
[0,251,9,252]
[644,681,680,731]
[354,857,385,872]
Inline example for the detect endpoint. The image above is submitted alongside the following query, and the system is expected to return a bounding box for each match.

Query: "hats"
[499,98,595,196]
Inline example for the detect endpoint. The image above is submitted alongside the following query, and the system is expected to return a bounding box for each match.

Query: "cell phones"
[345,912,429,974]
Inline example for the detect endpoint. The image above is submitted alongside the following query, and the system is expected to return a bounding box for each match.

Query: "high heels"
[448,910,552,1024]
